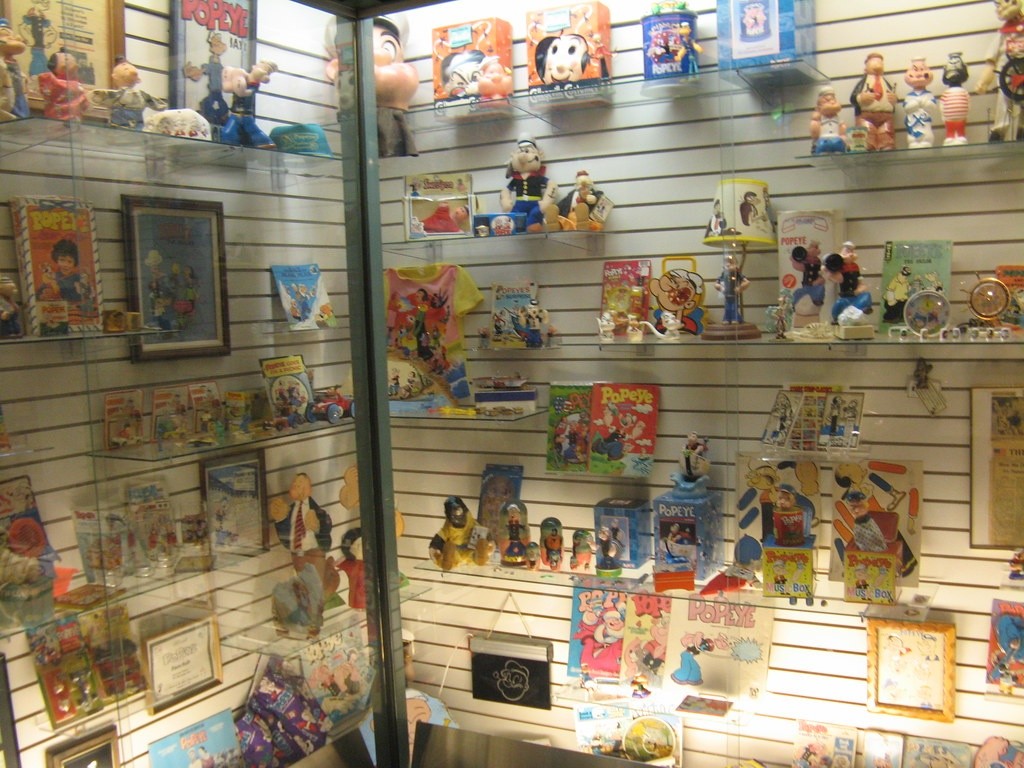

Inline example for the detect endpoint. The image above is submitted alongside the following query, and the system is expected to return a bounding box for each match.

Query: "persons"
[91,57,168,129]
[0,278,22,337]
[39,47,90,122]
[850,492,889,551]
[0,19,29,122]
[777,484,798,511]
[450,204,470,226]
[715,254,750,323]
[500,135,606,231]
[810,0,1024,156]
[599,527,616,568]
[223,60,279,148]
[676,24,705,80]
[517,301,549,347]
[822,242,874,326]
[683,432,704,464]
[325,14,419,157]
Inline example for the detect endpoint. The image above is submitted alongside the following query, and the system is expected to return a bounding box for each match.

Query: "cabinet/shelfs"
[0,60,1024,768]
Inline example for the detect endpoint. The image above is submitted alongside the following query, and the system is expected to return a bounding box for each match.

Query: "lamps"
[702,179,774,341]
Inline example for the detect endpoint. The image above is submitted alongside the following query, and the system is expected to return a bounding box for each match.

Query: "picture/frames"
[120,194,233,364]
[0,0,126,123]
[170,0,258,118]
[45,723,119,768]
[197,449,270,560]
[140,613,222,716]
[866,615,955,722]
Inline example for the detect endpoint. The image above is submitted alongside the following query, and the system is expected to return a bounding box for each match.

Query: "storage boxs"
[842,537,905,604]
[716,0,817,90]
[526,1,612,112]
[431,18,514,118]
[594,498,651,570]
[655,491,723,580]
[761,535,815,600]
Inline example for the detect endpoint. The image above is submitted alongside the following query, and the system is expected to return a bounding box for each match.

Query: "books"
[545,382,659,475]
[11,195,103,333]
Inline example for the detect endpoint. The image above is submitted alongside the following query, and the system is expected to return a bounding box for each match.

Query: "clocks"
[966,278,1011,322]
[904,289,951,337]
